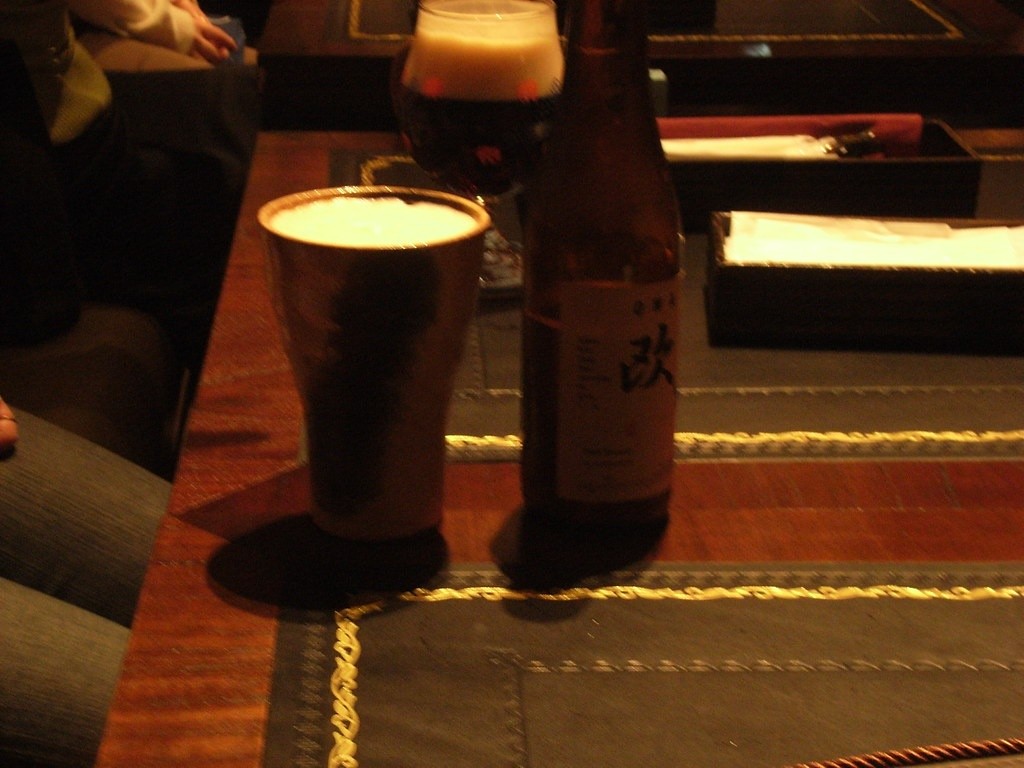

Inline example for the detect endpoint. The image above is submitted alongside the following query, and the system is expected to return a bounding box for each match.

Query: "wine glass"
[395,0,565,298]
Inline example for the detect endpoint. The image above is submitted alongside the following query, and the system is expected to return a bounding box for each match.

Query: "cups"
[258,185,492,543]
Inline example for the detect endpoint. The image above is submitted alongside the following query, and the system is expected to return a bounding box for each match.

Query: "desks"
[96,0,1024,768]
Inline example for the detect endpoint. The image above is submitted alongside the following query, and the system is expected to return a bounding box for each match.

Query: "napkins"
[722,210,1024,274]
[660,134,838,161]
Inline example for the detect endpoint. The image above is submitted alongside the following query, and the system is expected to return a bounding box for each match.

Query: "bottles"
[521,2,683,533]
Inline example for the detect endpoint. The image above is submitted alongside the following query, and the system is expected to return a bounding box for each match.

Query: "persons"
[0,0,255,347]
[0,405,171,768]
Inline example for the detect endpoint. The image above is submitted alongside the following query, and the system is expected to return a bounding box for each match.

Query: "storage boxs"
[705,211,1024,353]
[655,118,983,234]
